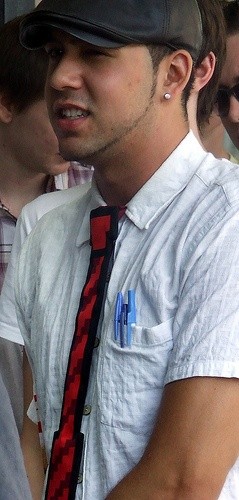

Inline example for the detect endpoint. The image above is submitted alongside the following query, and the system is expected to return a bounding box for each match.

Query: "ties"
[42,205,130,500]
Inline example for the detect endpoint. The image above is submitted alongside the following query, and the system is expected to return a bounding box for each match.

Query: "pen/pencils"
[114,291,122,342]
[120,304,128,348]
[128,289,136,326]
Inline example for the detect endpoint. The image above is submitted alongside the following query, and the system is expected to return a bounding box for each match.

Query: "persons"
[186,0,239,169]
[0,0,239,500]
[0,16,94,500]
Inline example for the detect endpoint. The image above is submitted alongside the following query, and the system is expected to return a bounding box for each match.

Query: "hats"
[18,0,202,62]
